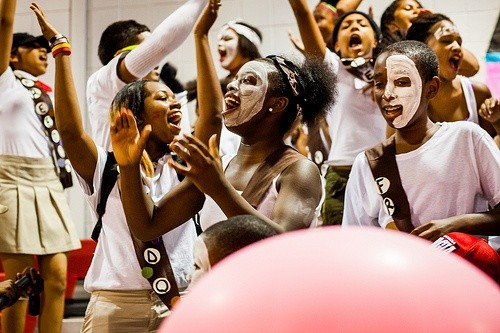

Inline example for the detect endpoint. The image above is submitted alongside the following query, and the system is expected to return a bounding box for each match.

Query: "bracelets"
[47,33,71,58]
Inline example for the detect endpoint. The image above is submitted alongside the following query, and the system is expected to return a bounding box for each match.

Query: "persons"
[386,13,497,139]
[86,0,210,152]
[186,214,278,292]
[110,55,339,243]
[341,40,500,243]
[288,0,388,228]
[0,0,83,333]
[30,0,224,333]
[159,0,500,182]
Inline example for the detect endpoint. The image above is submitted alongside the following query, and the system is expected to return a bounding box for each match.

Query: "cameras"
[16,266,44,316]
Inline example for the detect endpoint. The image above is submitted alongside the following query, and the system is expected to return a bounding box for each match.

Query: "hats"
[432,232,500,281]
[12,33,52,53]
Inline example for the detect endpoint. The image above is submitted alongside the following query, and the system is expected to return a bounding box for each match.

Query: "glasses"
[26,41,43,48]
